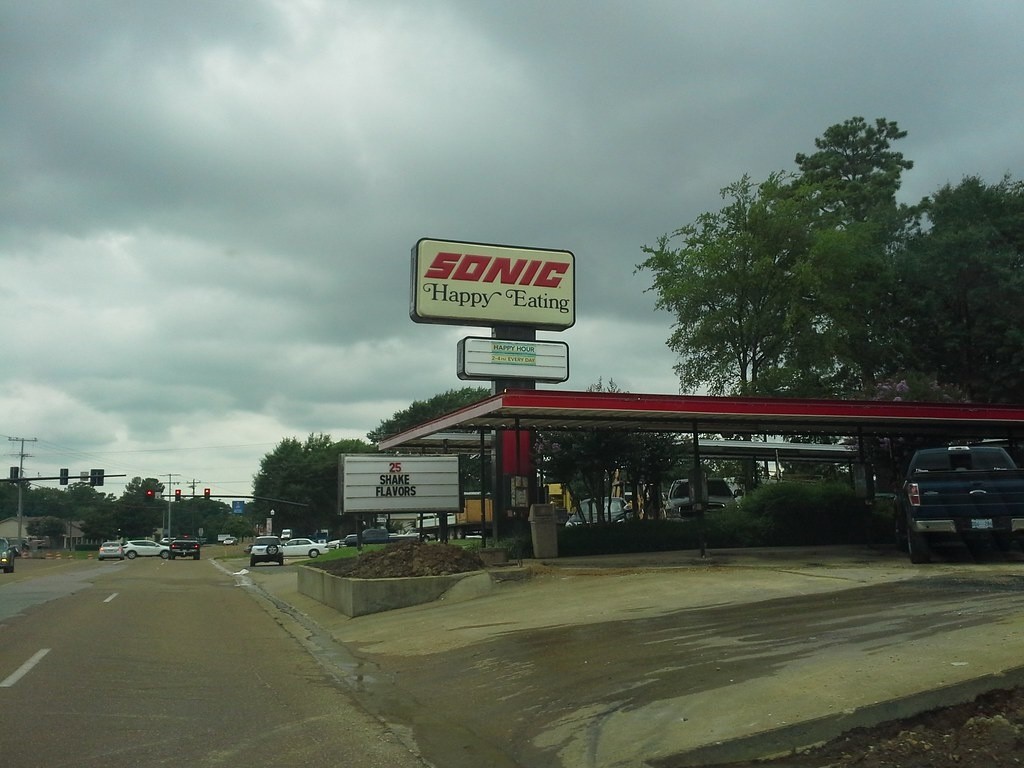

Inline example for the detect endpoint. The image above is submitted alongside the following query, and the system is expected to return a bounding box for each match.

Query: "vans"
[281,529,292,540]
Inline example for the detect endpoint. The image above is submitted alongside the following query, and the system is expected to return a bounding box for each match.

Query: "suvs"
[122,540,170,559]
[249,536,284,567]
[169,536,200,560]
[345,528,389,547]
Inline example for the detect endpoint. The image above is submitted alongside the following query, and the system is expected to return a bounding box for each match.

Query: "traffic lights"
[205,489,210,500]
[147,491,152,495]
[175,490,181,501]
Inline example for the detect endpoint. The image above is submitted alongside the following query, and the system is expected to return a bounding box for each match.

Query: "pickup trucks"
[890,445,1024,565]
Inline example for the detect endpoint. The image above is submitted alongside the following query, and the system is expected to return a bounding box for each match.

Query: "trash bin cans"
[528,502,559,559]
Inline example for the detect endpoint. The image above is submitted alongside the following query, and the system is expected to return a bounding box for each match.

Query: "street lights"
[271,509,275,536]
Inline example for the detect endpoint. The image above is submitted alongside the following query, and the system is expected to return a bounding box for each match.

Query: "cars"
[661,477,743,518]
[160,538,176,543]
[283,538,329,558]
[223,537,238,546]
[98,542,124,560]
[0,538,16,573]
[564,497,631,527]
